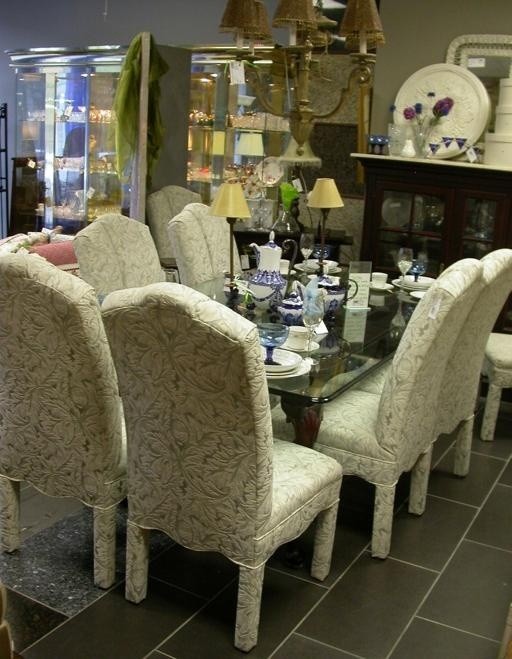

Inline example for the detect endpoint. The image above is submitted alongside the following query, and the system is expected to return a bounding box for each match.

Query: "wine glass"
[303,293,325,362]
[397,248,413,293]
[390,294,406,337]
[411,259,425,281]
[257,323,290,364]
[296,233,331,280]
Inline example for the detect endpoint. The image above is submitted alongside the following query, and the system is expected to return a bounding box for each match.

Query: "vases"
[408,124,435,159]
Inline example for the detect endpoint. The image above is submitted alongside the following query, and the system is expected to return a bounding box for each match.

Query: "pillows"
[0,228,75,266]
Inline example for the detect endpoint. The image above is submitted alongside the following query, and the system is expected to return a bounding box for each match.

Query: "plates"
[380,196,412,227]
[280,339,320,353]
[392,275,437,298]
[369,282,395,292]
[256,156,284,184]
[261,377,311,389]
[263,349,311,377]
[295,258,342,274]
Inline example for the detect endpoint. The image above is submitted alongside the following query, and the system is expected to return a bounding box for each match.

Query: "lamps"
[207,183,252,299]
[306,178,345,258]
[217,0,385,156]
[277,136,324,230]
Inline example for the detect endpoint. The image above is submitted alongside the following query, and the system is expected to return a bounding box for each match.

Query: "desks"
[233,220,354,270]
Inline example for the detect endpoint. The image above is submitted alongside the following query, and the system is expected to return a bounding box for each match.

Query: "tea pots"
[247,231,289,310]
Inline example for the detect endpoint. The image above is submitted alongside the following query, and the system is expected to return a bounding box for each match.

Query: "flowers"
[390,90,455,124]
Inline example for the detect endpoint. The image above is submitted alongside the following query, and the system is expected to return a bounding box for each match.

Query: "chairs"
[0,253,129,590]
[363,248,512,518]
[480,333,512,443]
[146,187,203,269]
[271,258,484,563]
[100,280,342,651]
[73,212,167,301]
[167,200,244,287]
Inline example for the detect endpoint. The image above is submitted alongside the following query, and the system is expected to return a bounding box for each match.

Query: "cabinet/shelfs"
[4,45,292,233]
[351,152,512,332]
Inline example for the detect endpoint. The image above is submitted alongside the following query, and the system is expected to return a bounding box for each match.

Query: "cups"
[371,272,387,288]
[244,199,279,230]
[288,326,316,348]
[386,123,407,156]
[369,295,385,306]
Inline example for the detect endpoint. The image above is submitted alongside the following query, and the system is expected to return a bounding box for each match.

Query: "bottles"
[276,293,304,323]
[272,210,299,236]
[314,319,342,360]
[402,135,415,156]
[313,276,347,316]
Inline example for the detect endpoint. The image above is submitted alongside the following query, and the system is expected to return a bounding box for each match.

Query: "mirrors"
[445,33,512,151]
